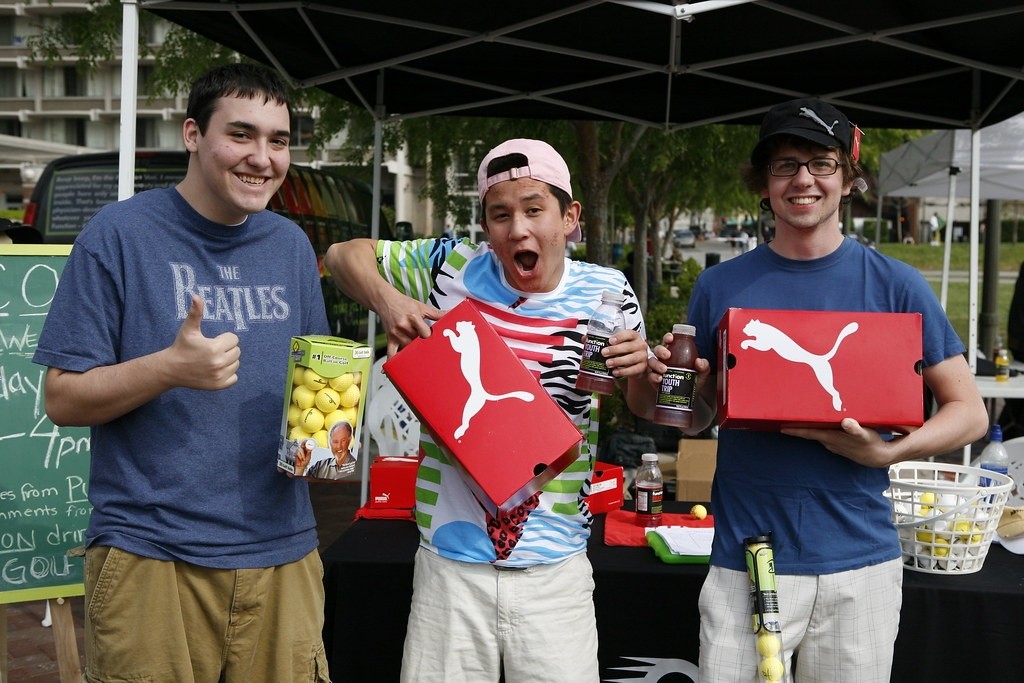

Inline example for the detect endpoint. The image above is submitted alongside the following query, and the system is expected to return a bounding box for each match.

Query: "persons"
[929,211,939,246]
[324,136,659,683]
[764,224,773,243]
[293,421,357,479]
[741,228,749,254]
[748,231,757,250]
[644,98,992,682]
[44,61,335,683]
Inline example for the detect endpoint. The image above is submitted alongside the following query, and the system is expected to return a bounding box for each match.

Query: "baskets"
[883,460,1013,574]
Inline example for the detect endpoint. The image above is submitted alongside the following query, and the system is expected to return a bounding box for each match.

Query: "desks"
[317,482,1024,683]
[962,352,1024,442]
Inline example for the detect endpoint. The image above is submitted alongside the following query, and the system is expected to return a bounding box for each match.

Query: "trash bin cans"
[705,252,721,269]
[612,243,623,264]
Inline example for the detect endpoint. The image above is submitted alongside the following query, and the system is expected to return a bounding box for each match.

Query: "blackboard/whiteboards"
[0,243,92,605]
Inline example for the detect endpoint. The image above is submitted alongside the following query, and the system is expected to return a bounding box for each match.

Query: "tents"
[875,114,1023,465]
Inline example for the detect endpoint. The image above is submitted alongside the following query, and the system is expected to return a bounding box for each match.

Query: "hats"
[751,99,851,168]
[477,139,583,244]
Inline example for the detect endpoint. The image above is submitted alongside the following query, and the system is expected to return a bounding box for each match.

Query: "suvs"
[21,148,414,338]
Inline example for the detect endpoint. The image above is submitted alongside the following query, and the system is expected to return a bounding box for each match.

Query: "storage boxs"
[588,462,625,515]
[678,437,717,502]
[715,308,923,431]
[382,298,585,523]
[371,456,420,508]
[277,334,375,480]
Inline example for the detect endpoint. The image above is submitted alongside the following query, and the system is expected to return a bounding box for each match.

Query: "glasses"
[763,157,846,176]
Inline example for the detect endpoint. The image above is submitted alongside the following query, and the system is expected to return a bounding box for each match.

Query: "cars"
[0,135,102,227]
[669,229,697,249]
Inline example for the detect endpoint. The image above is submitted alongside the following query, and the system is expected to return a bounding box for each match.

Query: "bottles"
[996,350,1009,382]
[979,424,1009,513]
[993,339,1005,377]
[576,290,626,397]
[653,323,697,429]
[634,453,663,527]
